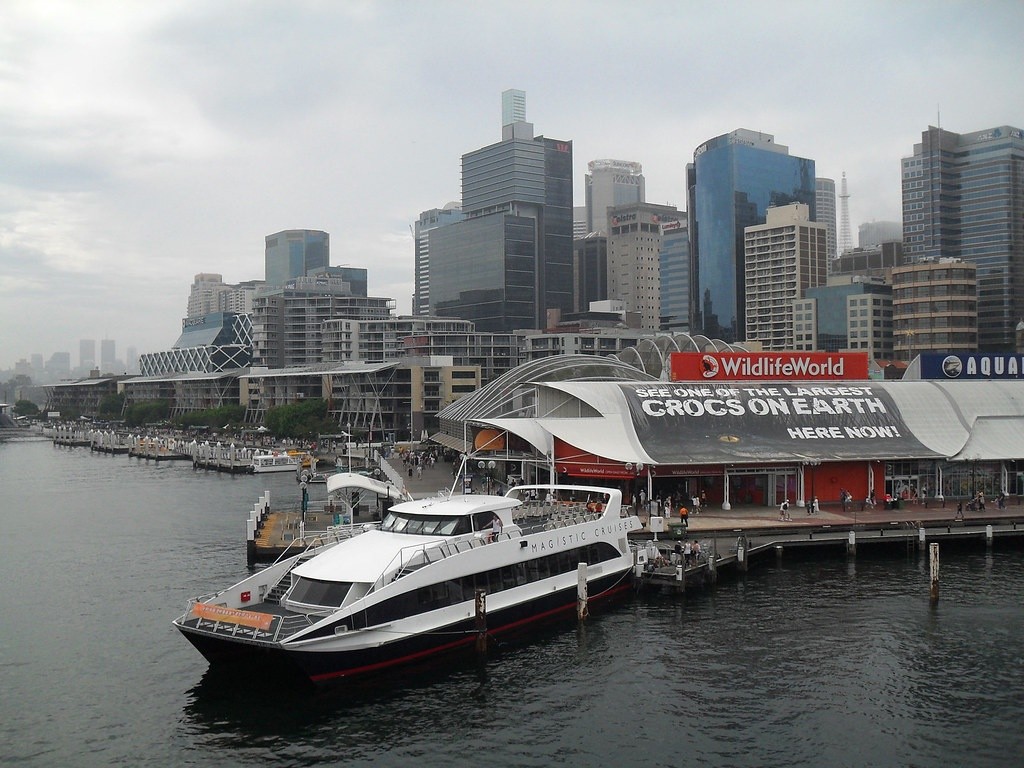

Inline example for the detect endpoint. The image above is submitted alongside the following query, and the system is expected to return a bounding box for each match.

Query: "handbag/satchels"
[980,497,985,504]
[813,503,816,506]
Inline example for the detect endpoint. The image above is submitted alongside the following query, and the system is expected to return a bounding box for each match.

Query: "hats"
[815,496,818,498]
[678,541,681,544]
[787,499,789,502]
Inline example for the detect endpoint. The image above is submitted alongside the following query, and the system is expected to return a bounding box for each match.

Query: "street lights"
[346,422,352,476]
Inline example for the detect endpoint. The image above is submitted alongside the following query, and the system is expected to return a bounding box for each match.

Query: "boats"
[174,484,647,691]
[248,453,303,471]
[287,450,320,466]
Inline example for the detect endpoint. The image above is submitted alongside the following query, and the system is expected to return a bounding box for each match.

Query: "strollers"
[967,497,979,511]
[866,498,874,509]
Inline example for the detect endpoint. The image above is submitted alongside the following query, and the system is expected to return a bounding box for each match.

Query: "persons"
[211,430,1008,527]
[482,515,504,542]
[674,540,684,565]
[692,540,701,564]
[682,539,693,567]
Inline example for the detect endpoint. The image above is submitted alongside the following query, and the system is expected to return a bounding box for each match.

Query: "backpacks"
[783,504,787,510]
